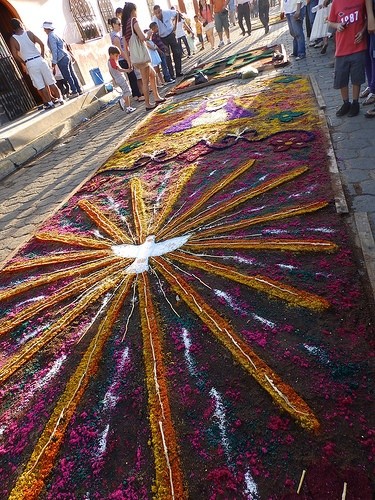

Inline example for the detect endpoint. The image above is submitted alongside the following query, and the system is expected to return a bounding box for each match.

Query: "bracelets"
[52,67,55,69]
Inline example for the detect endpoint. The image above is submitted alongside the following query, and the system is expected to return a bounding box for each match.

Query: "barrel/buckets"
[89,68,104,85]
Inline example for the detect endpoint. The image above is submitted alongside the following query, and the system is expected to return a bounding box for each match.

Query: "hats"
[41,21,55,30]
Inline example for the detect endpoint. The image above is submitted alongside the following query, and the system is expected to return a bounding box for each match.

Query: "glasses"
[116,23,122,26]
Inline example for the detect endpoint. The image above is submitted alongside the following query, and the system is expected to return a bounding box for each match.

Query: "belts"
[24,55,41,63]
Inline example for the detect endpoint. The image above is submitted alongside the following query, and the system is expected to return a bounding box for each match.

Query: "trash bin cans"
[88,67,104,85]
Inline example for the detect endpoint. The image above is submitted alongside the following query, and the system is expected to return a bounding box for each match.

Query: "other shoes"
[181,50,196,58]
[360,87,371,98]
[362,93,375,106]
[347,102,361,117]
[265,25,269,34]
[218,38,231,47]
[126,107,137,114]
[145,97,166,112]
[200,47,206,51]
[309,41,317,47]
[365,107,375,117]
[240,31,251,36]
[43,91,83,110]
[289,51,306,61]
[320,44,328,54]
[118,99,126,111]
[313,41,323,48]
[134,96,145,102]
[156,72,184,88]
[336,101,351,116]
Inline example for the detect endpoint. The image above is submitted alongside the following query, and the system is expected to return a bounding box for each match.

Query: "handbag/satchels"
[202,20,208,27]
[127,17,153,66]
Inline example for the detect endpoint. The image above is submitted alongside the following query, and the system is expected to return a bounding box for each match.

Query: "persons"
[305,0,335,54]
[108,17,145,102]
[228,0,236,26]
[48,60,69,97]
[194,15,204,51]
[235,0,251,36]
[259,0,269,34]
[170,7,194,57]
[328,0,375,117]
[108,47,136,114]
[198,0,215,50]
[9,18,65,110]
[143,21,175,88]
[123,2,166,111]
[41,21,83,99]
[210,0,230,47]
[280,0,307,61]
[149,5,184,79]
[116,7,151,96]
